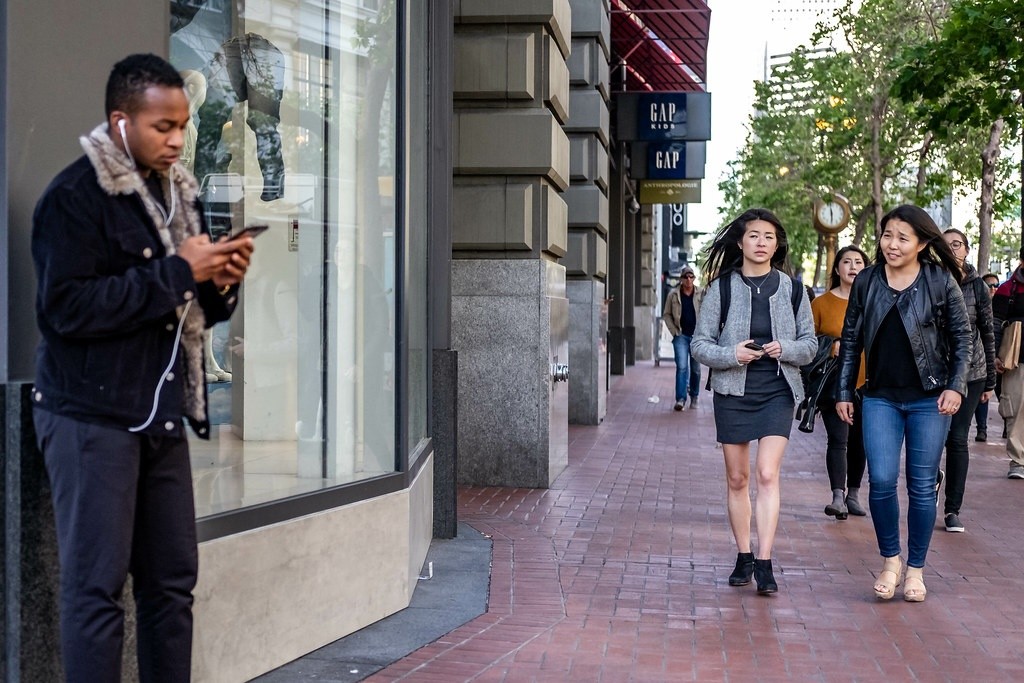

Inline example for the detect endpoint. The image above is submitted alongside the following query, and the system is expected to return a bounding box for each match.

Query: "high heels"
[873,557,902,600]
[903,569,927,602]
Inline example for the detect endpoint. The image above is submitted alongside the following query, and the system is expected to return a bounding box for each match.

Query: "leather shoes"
[975,427,988,442]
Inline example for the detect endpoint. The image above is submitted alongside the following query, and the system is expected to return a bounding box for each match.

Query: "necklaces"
[741,265,774,294]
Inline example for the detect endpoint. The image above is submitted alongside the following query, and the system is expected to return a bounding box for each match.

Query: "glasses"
[949,240,965,249]
[683,274,694,278]
[986,282,1000,288]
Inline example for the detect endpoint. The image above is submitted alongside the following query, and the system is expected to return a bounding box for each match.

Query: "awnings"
[610,0,712,91]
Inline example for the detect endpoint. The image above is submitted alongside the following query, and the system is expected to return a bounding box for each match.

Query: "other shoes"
[729,552,754,586]
[753,558,779,592]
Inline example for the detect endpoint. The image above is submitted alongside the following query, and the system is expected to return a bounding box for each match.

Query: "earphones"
[118,118,126,139]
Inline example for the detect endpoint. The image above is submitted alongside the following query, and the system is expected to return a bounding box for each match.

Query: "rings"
[952,406,958,410]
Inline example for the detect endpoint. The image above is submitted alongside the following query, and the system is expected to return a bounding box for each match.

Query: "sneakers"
[1006,461,1024,480]
[674,398,684,411]
[943,512,966,532]
[690,397,700,409]
[934,471,945,506]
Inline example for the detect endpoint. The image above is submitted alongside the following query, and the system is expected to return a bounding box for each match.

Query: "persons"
[660,268,672,320]
[935,229,996,532]
[992,246,1024,478]
[809,245,874,519]
[835,205,973,601]
[691,207,818,594]
[31,52,253,683]
[663,265,707,412]
[975,273,1008,442]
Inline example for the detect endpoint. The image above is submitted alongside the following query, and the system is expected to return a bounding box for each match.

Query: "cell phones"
[225,225,269,244]
[745,343,765,351]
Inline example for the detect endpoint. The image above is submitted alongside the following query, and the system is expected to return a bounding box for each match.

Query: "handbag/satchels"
[998,320,1022,370]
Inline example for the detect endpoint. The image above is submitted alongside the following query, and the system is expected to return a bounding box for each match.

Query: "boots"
[823,489,849,521]
[845,488,866,516]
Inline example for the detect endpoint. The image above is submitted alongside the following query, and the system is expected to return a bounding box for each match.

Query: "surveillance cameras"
[629,197,640,214]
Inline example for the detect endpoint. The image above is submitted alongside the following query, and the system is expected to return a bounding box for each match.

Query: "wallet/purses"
[744,342,764,352]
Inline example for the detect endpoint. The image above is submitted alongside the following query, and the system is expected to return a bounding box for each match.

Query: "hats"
[680,267,694,278]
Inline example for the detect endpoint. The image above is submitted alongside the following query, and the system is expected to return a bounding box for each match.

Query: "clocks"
[819,202,845,226]
